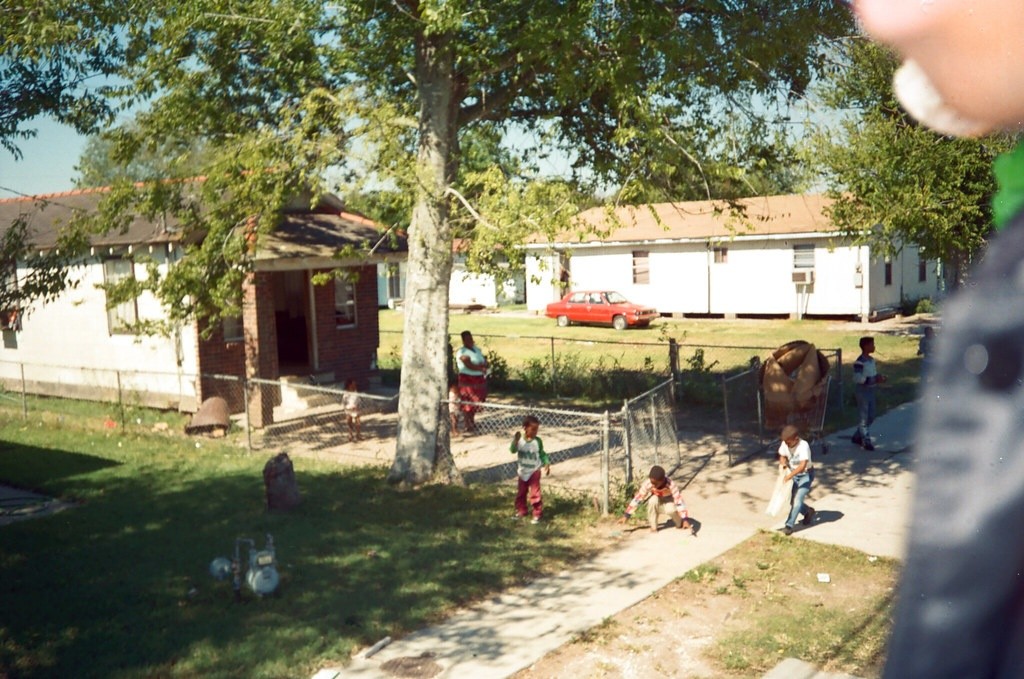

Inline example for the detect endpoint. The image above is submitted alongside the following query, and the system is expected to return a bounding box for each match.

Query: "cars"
[544,288,660,331]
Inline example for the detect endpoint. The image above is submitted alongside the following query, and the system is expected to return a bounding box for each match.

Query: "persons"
[618,465,692,533]
[342,380,364,441]
[779,425,816,536]
[510,416,549,527]
[852,336,886,452]
[917,327,938,366]
[855,0,1024,678]
[455,331,487,433]
[450,384,462,436]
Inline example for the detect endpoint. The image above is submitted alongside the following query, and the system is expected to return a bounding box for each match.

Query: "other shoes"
[463,431,475,436]
[864,443,874,450]
[803,508,815,525]
[852,436,862,445]
[532,517,542,524]
[511,513,528,519]
[784,526,793,536]
